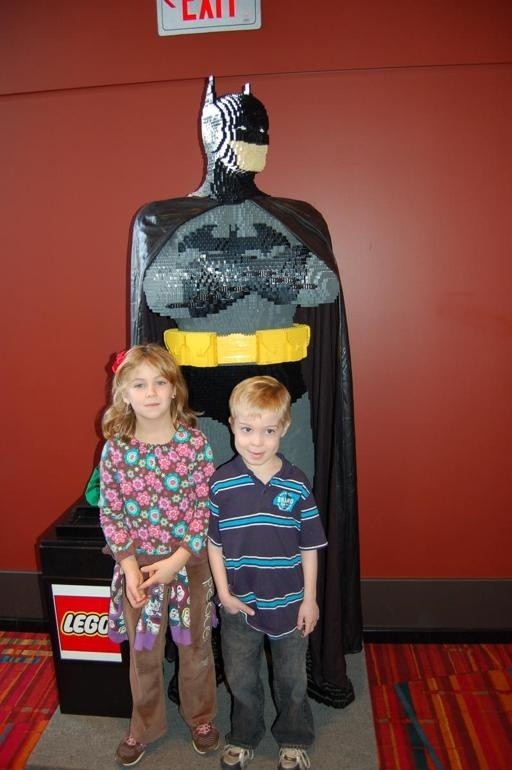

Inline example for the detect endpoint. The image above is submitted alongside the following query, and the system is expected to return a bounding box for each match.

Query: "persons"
[204,372,331,770]
[84,342,221,768]
[126,75,362,708]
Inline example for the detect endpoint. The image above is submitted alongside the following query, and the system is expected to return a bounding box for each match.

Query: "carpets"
[24,641,378,769]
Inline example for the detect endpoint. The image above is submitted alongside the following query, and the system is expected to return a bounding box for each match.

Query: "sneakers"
[190,721,220,756]
[114,736,146,766]
[277,746,313,769]
[221,743,256,770]
